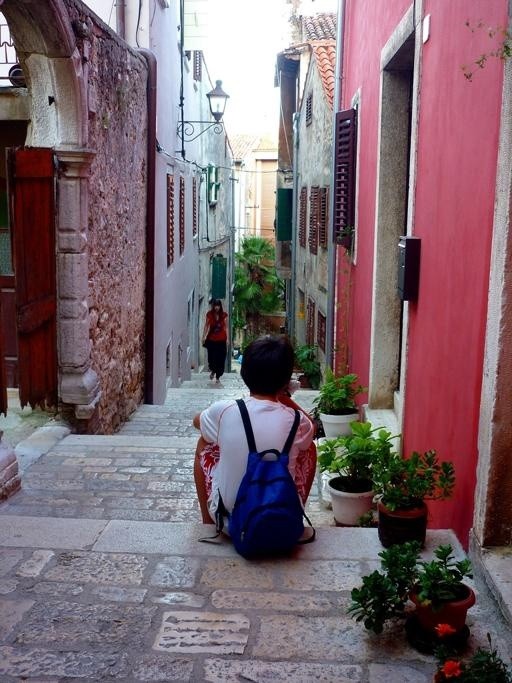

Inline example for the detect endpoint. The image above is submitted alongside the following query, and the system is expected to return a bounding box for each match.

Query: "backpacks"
[197,399,316,563]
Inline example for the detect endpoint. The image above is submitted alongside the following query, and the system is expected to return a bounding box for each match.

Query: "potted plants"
[371,448,457,549]
[294,344,322,390]
[309,365,369,438]
[315,420,402,526]
[345,539,476,636]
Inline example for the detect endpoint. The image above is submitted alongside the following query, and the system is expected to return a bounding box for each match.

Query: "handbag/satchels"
[202,320,220,348]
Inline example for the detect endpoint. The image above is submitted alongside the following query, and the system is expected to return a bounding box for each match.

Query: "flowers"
[435,624,456,636]
[440,660,464,677]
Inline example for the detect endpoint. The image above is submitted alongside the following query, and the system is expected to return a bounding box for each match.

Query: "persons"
[194,336,317,537]
[202,299,229,385]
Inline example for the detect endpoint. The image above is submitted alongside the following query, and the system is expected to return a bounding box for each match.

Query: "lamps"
[176,80,231,142]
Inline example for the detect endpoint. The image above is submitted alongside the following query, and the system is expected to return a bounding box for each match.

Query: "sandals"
[216,377,220,384]
[210,371,215,380]
